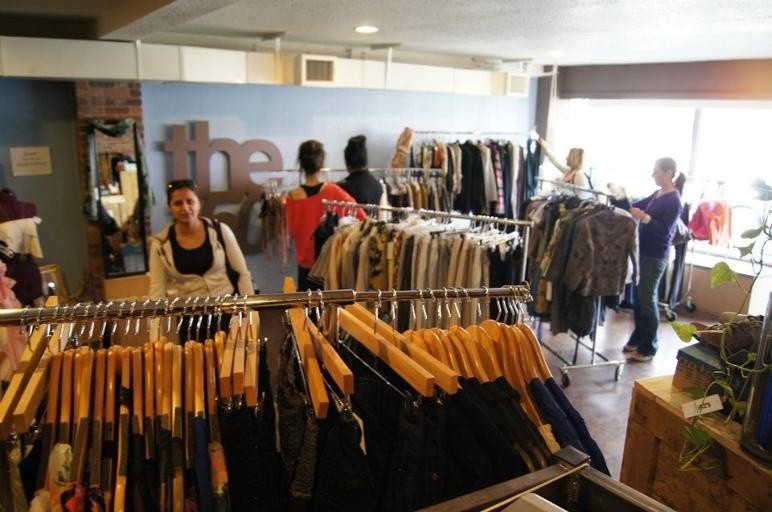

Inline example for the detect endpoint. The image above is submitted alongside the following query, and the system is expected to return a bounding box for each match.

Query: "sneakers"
[623,344,637,351]
[632,353,653,361]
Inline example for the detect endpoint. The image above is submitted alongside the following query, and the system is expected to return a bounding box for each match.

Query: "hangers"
[370,167,444,182]
[409,129,531,157]
[260,171,280,199]
[535,176,612,209]
[1,280,563,512]
[322,197,522,252]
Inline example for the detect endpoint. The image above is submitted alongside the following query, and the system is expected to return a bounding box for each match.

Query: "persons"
[0,188,43,308]
[611,156,686,362]
[538,138,591,197]
[286,135,386,290]
[148,178,256,347]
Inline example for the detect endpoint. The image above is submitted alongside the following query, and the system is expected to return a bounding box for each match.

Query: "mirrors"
[91,116,149,278]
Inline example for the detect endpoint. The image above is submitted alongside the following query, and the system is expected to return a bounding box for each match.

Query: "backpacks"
[687,202,712,241]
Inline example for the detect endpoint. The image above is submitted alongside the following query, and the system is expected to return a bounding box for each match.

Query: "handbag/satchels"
[671,217,692,246]
[227,269,260,295]
